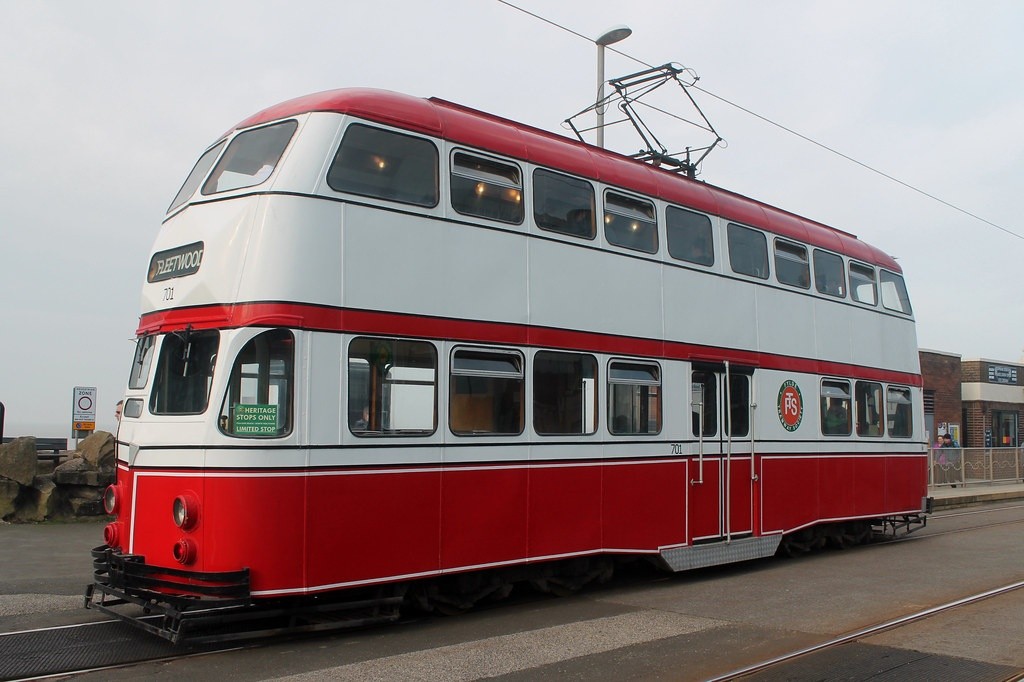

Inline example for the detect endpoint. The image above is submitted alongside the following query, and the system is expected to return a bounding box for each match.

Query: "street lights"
[593,25,634,150]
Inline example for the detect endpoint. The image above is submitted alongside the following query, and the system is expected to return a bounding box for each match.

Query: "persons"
[1020,442,1024,483]
[932,435,947,486]
[114,400,122,421]
[354,406,369,429]
[937,433,965,488]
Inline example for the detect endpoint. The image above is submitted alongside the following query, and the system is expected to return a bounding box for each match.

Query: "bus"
[83,85,933,654]
